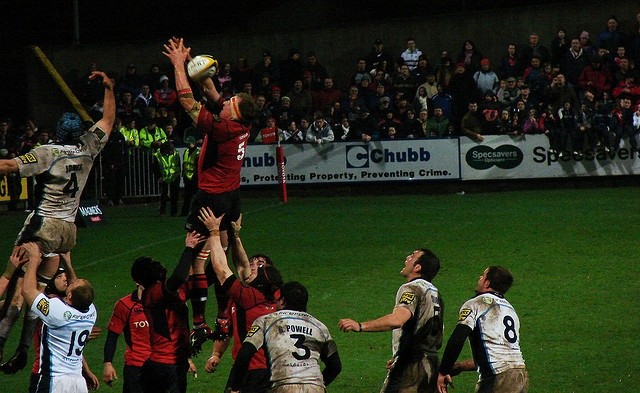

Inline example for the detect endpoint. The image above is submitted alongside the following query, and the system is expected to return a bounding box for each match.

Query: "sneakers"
[117,199,125,206]
[157,211,165,217]
[178,211,189,218]
[208,312,230,343]
[1,349,28,374]
[169,212,177,218]
[107,200,113,206]
[188,322,213,358]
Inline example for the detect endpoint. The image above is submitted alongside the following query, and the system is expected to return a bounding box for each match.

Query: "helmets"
[55,112,83,144]
[45,267,69,296]
[250,264,282,301]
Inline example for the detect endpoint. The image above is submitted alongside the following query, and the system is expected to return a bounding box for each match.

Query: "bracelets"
[222,247,228,252]
[196,249,211,261]
[359,323,362,332]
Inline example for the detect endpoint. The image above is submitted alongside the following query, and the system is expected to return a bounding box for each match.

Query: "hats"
[480,58,489,66]
[183,135,197,147]
[375,38,382,45]
[271,87,280,94]
[302,70,311,78]
[159,76,169,83]
[505,77,515,83]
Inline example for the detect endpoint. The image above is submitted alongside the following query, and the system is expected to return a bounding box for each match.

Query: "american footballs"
[187,54,219,81]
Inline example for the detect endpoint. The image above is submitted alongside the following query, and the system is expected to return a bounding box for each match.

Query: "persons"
[0,245,30,310]
[160,35,260,356]
[151,141,181,218]
[438,266,530,393]
[183,119,204,147]
[246,15,640,158]
[0,72,117,375]
[231,281,341,393]
[0,122,21,214]
[131,229,229,393]
[87,92,103,121]
[101,118,128,207]
[169,116,179,131]
[205,253,283,373]
[152,75,177,118]
[20,241,98,393]
[78,62,102,108]
[338,248,444,393]
[142,65,167,93]
[126,62,140,92]
[30,131,56,212]
[118,79,128,92]
[179,136,201,216]
[117,90,133,118]
[158,105,169,126]
[120,118,140,203]
[143,107,156,120]
[28,266,103,393]
[24,121,31,129]
[201,58,246,99]
[29,116,36,127]
[198,206,283,393]
[165,124,182,147]
[102,282,152,393]
[11,127,38,212]
[6,118,15,133]
[103,71,117,97]
[139,119,167,203]
[135,84,153,128]
